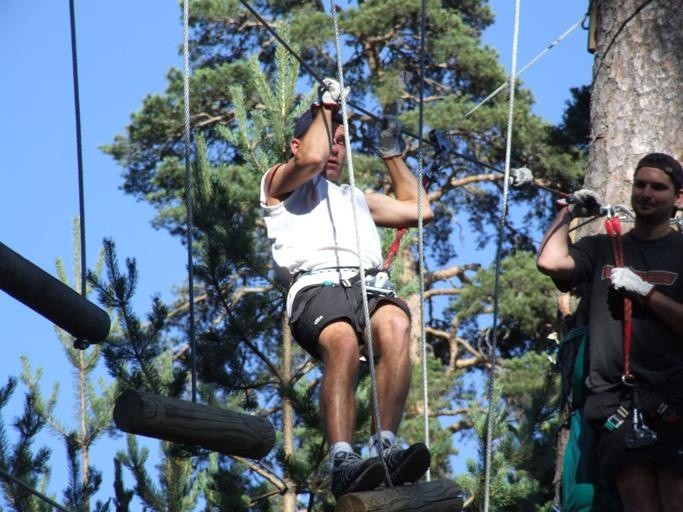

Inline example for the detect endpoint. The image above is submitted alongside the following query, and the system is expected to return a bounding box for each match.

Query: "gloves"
[555,188,603,221]
[378,116,403,160]
[311,77,351,112]
[610,264,655,298]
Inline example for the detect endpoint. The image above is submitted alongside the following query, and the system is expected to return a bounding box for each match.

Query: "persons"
[538,151,682,511]
[259,76,437,503]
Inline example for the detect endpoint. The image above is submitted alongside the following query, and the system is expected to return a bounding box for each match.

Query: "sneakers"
[372,434,430,489]
[330,447,386,500]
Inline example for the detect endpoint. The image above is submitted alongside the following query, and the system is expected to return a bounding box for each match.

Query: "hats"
[294,106,353,144]
[633,153,683,218]
